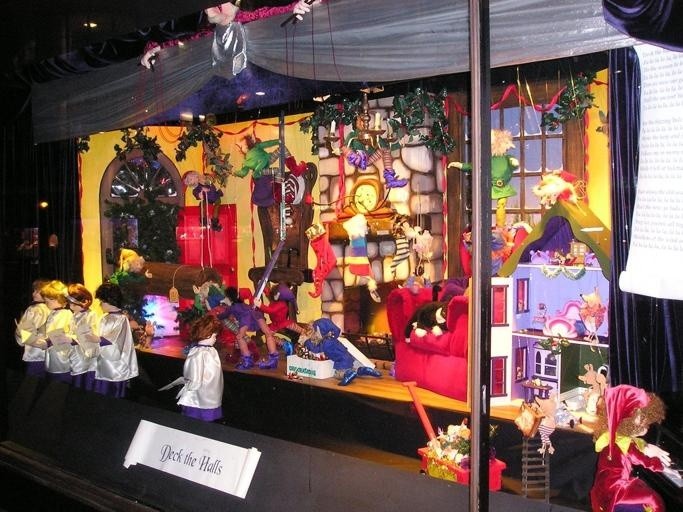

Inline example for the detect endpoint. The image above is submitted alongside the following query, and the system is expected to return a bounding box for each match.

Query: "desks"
[133,337,599,503]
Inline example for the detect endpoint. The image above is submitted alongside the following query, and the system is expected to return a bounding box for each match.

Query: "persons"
[181,170,224,232]
[346,129,411,188]
[109,247,153,288]
[16,279,98,391]
[298,318,381,387]
[192,269,306,369]
[85,282,139,398]
[176,314,224,422]
[228,133,306,183]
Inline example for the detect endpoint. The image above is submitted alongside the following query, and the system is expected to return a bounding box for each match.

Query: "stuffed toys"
[531,394,558,455]
[577,362,609,416]
[591,383,673,511]
[137,0,315,123]
[445,125,611,279]
[403,284,453,345]
